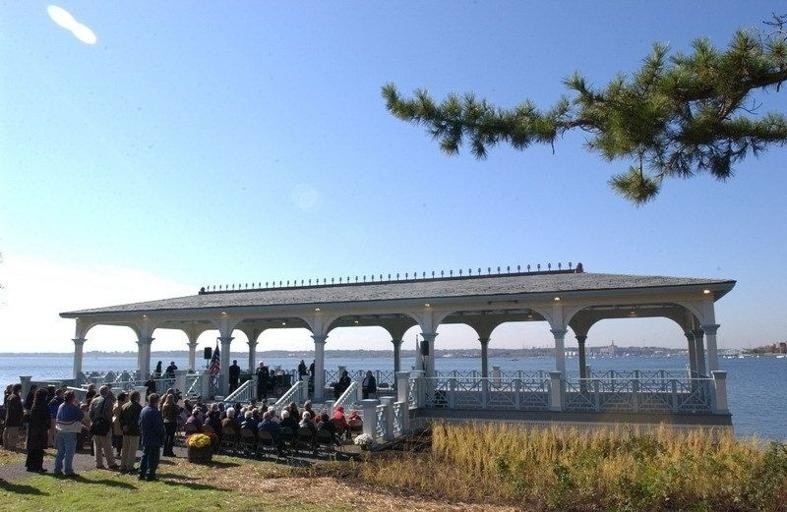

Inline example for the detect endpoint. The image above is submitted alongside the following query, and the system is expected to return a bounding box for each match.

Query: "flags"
[208,347,220,380]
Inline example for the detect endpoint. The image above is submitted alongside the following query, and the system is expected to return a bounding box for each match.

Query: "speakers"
[420,341,429,355]
[204,347,212,359]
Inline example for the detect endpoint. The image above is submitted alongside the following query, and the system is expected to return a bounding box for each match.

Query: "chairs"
[184,419,362,463]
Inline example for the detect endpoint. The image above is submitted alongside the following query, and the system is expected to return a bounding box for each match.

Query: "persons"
[178,393,363,456]
[155,359,378,400]
[1,378,184,482]
